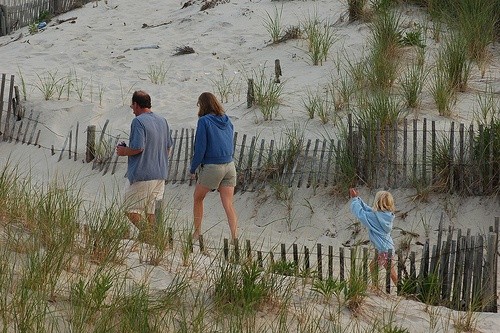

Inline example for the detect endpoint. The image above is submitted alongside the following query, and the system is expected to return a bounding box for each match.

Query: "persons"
[349,188,402,292]
[116,90,174,244]
[186,92,240,246]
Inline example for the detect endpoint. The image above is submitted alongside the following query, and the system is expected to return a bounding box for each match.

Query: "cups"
[118,141,128,148]
